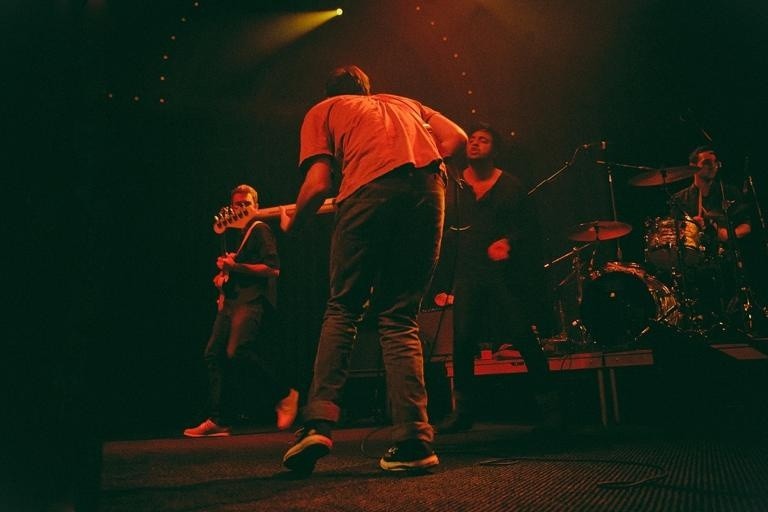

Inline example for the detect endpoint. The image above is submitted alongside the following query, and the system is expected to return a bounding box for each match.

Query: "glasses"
[702,158,723,170]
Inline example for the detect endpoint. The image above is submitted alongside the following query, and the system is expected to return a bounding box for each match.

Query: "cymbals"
[628,166,701,186]
[562,220,632,242]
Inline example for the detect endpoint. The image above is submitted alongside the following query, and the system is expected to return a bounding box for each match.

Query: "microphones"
[578,141,607,152]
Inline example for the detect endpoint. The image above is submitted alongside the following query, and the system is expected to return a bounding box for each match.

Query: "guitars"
[213,197,336,234]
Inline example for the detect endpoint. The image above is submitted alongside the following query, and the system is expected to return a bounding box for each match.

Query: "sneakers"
[281,420,335,473]
[378,434,440,472]
[183,417,230,437]
[274,388,299,431]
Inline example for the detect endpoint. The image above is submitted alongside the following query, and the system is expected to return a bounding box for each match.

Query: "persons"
[438,122,566,436]
[668,145,751,321]
[183,183,299,437]
[278,64,469,473]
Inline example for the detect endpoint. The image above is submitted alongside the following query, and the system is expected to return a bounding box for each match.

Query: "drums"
[575,261,680,345]
[643,215,699,262]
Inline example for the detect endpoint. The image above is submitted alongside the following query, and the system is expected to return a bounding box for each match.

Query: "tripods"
[633,183,768,347]
